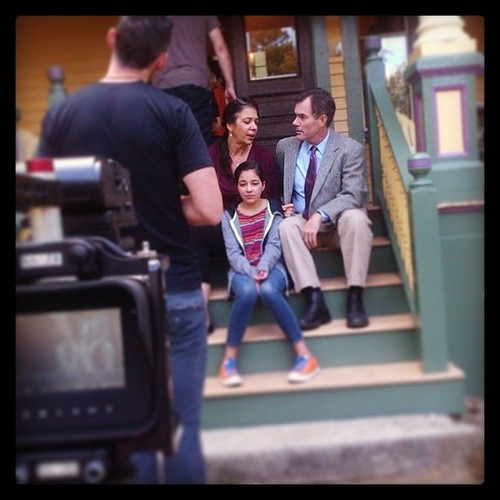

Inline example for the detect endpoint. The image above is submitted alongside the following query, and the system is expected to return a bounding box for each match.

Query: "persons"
[40,17,224,484]
[219,160,320,387]
[149,14,244,144]
[275,87,374,331]
[199,98,282,334]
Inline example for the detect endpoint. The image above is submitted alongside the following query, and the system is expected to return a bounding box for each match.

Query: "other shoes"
[216,360,242,386]
[288,357,319,381]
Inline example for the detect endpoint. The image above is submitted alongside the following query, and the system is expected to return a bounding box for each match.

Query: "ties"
[302,146,318,222]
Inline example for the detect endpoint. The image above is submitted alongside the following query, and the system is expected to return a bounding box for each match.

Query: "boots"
[296,288,331,329]
[345,285,369,327]
